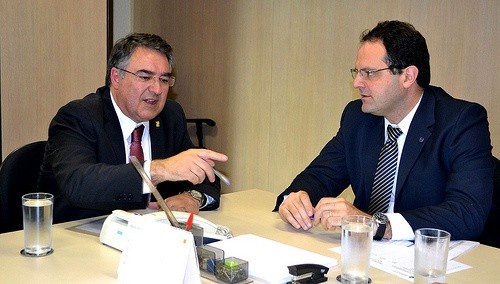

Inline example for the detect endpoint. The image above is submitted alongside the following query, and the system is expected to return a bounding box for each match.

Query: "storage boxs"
[213,257,249,283]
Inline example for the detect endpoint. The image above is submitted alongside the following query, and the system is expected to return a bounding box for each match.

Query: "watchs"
[372,212,389,242]
[187,189,204,208]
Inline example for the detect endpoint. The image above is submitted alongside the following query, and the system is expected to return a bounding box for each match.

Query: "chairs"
[0,139,49,234]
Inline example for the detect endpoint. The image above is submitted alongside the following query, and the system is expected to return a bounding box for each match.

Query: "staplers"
[285,264,330,284]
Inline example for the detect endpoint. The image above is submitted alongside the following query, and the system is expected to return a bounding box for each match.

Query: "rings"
[329,210,332,217]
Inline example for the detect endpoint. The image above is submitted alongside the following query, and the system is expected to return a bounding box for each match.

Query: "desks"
[0,188,500,284]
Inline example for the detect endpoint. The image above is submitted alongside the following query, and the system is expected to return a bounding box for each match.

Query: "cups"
[21,192,54,256]
[414,228,451,284]
[340,214,374,284]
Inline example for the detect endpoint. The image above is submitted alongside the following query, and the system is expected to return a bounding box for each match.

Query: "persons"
[272,19,495,241]
[31,32,229,225]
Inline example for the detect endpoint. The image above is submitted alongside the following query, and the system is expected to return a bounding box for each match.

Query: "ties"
[369,126,403,216]
[128,125,145,208]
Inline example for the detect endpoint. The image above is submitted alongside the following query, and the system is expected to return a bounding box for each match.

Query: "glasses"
[351,67,393,80]
[114,66,176,87]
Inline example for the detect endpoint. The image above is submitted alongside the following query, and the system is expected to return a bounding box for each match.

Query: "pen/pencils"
[185,211,194,233]
[310,214,322,220]
[211,167,231,186]
